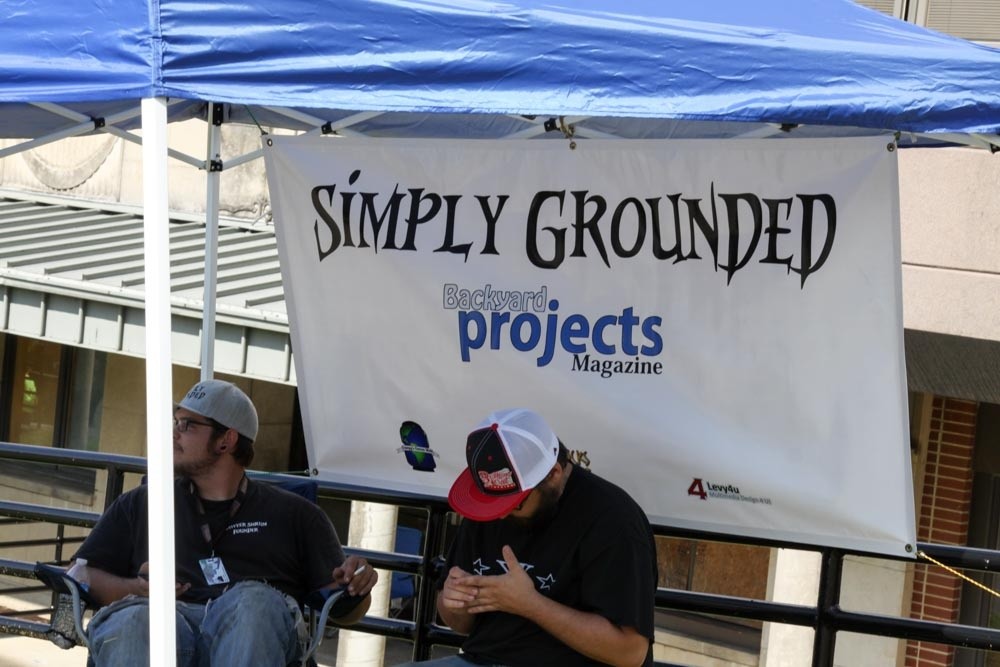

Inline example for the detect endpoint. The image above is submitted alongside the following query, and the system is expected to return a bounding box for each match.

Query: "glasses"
[513,491,536,511]
[173,416,216,433]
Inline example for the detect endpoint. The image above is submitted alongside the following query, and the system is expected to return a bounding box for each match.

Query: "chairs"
[35,474,454,667]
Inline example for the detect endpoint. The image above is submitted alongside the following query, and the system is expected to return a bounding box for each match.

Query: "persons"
[387,407,655,667]
[66,378,379,667]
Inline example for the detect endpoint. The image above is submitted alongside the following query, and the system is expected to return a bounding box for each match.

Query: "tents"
[1,0,999,667]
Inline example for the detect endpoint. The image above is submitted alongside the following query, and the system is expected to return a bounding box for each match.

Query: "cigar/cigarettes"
[354,565,367,577]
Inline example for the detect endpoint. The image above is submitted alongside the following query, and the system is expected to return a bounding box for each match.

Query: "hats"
[173,379,258,441]
[448,408,557,521]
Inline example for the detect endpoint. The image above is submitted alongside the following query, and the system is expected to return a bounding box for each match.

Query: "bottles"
[49,558,91,648]
